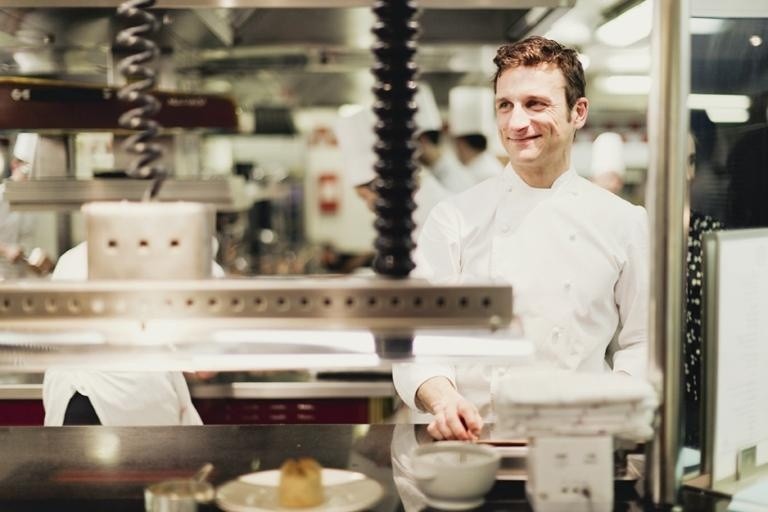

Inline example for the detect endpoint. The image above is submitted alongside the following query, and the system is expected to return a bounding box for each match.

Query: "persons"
[678,125,723,441]
[592,166,625,196]
[1,153,62,284]
[389,34,667,444]
[446,132,506,193]
[37,242,232,428]
[415,130,475,199]
[355,163,447,235]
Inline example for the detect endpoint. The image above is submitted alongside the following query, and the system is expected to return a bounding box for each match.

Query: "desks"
[2,417,688,512]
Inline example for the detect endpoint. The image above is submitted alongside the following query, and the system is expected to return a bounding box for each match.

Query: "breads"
[279,456,322,511]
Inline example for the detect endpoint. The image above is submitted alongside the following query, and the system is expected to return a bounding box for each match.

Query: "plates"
[214,468,384,511]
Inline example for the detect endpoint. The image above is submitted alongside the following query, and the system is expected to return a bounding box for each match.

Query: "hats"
[337,99,382,188]
[589,133,624,179]
[450,86,495,149]
[410,83,443,138]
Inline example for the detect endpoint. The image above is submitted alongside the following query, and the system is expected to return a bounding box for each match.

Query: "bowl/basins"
[410,441,501,510]
[143,479,215,511]
